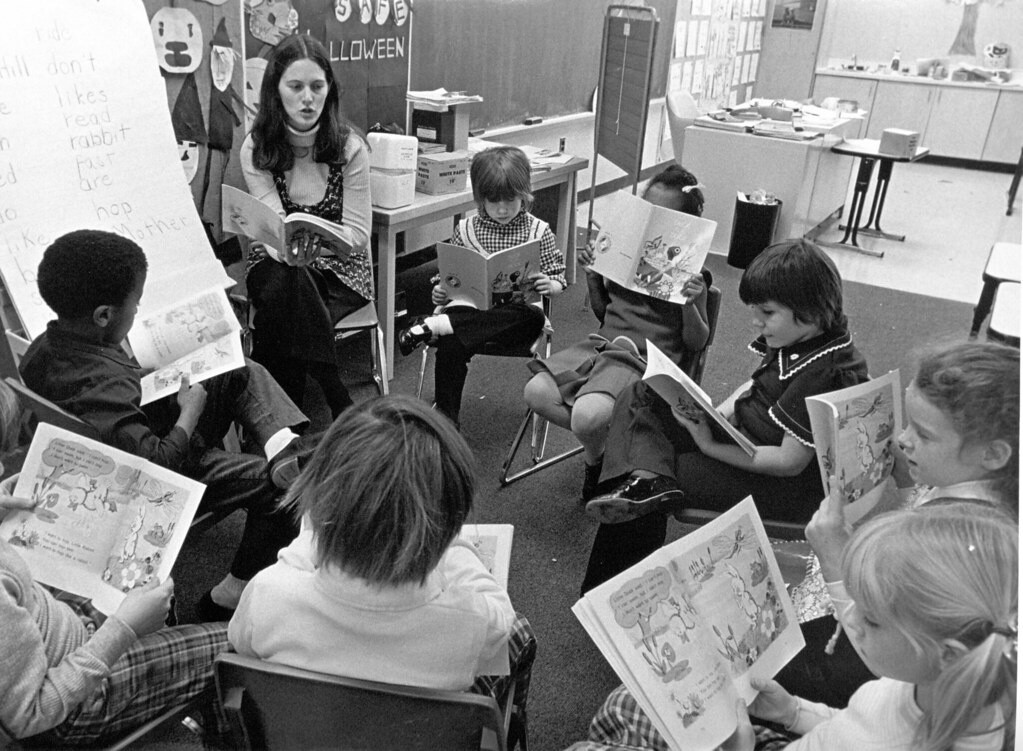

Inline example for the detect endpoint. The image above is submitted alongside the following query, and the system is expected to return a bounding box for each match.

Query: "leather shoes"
[399,315,432,356]
[585,474,684,524]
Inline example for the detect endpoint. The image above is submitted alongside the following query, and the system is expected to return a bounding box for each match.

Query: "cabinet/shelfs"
[811,74,1023,173]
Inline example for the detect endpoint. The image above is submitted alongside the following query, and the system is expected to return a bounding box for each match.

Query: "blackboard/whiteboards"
[409,1,677,139]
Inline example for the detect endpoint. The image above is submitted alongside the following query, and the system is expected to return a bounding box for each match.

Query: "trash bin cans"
[727,194,783,270]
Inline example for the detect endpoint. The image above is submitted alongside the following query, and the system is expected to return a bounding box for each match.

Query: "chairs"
[1,279,875,751]
[665,89,702,163]
[968,242,1022,351]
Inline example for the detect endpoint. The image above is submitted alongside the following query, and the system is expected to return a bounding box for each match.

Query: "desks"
[678,110,863,259]
[811,138,930,258]
[370,140,588,380]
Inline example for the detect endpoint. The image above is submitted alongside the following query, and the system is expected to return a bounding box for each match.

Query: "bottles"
[792,107,802,129]
[891,47,901,72]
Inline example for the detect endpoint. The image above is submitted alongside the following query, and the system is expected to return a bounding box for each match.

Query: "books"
[121,285,243,407]
[572,495,808,751]
[220,185,352,263]
[807,370,904,524]
[440,239,544,309]
[295,507,514,674]
[584,187,717,306]
[642,339,760,459]
[0,422,206,619]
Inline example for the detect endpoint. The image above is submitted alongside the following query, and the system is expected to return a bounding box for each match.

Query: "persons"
[237,38,375,439]
[563,237,1021,750]
[0,228,535,751]
[523,167,727,502]
[401,149,568,435]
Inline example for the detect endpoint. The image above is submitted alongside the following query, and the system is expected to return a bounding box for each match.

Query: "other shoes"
[194,588,235,621]
[583,460,600,501]
[266,429,327,489]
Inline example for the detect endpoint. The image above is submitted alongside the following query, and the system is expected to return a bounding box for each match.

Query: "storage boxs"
[415,151,469,196]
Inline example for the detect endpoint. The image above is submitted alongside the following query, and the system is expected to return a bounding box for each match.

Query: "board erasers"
[525,117,543,124]
[468,129,485,137]
